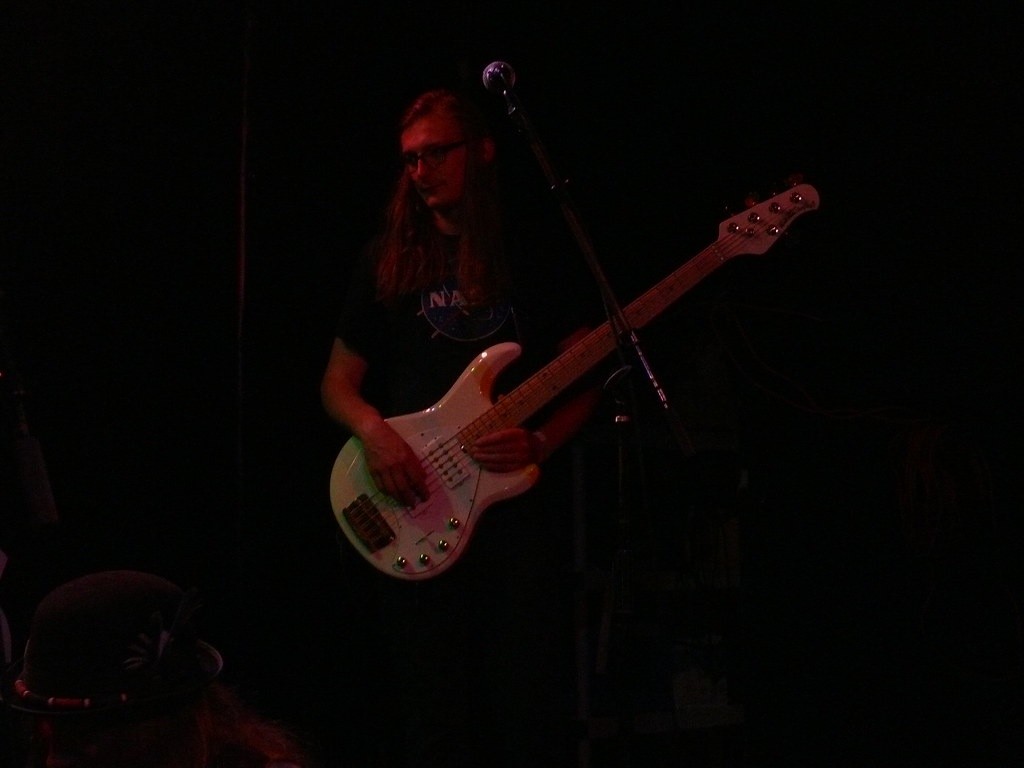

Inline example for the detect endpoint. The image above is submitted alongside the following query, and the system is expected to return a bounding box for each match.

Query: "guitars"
[330,178,821,580]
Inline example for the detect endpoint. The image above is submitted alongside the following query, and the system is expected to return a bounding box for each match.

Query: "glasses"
[403,139,473,173]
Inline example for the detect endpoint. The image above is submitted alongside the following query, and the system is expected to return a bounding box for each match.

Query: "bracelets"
[530,429,550,465]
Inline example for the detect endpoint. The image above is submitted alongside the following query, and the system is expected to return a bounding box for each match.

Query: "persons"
[0,567,306,768]
[315,91,604,768]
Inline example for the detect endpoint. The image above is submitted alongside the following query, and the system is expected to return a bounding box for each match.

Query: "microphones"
[481,61,516,93]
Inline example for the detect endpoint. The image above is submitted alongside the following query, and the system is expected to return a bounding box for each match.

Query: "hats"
[2,571,224,721]
